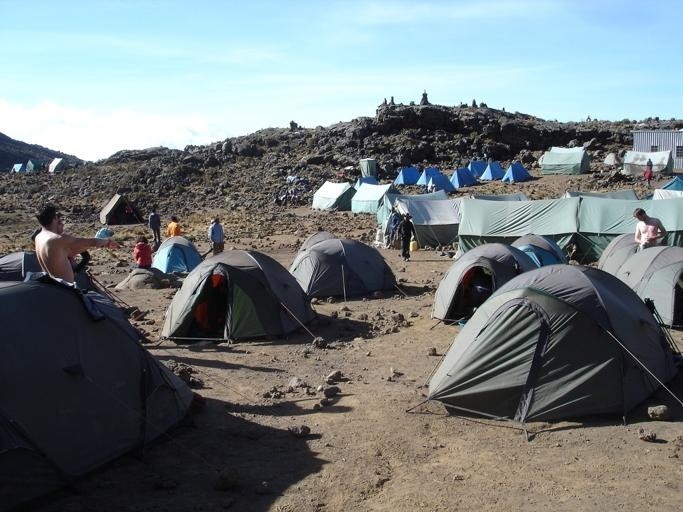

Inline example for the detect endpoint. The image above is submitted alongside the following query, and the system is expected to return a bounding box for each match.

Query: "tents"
[154,250,316,349]
[25,159,47,172]
[48,157,72,174]
[406,264,679,441]
[0,250,113,301]
[151,236,202,274]
[0,282,193,512]
[290,145,682,328]
[99,194,143,225]
[12,163,25,173]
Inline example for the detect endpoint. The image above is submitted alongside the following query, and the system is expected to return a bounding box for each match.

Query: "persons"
[31,212,89,273]
[35,203,120,284]
[208,217,225,255]
[166,216,180,237]
[149,208,160,242]
[133,236,153,268]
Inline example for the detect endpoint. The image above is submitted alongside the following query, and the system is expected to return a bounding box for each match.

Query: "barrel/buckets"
[410,240,418,252]
